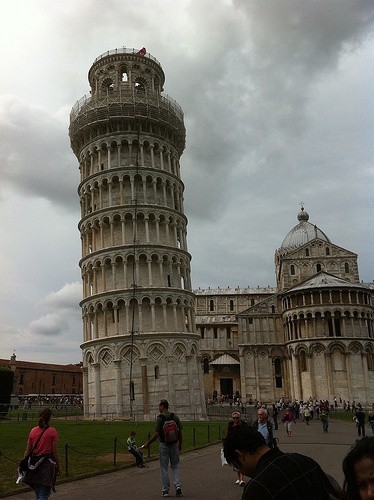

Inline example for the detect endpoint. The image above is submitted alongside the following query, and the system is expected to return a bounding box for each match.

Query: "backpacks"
[157,413,179,445]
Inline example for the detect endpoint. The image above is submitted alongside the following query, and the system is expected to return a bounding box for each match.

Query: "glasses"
[232,417,239,419]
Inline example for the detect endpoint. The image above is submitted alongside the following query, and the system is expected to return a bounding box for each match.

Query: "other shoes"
[239,481,245,486]
[235,480,240,483]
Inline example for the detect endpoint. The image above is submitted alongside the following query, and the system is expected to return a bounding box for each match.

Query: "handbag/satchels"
[19,453,31,472]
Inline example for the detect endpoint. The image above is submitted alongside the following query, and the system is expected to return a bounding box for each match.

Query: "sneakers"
[161,486,169,497]
[176,487,183,495]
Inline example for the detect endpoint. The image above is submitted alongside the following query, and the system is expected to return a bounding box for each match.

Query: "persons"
[222,424,344,500]
[17,395,83,421]
[126,431,145,468]
[342,436,374,500]
[206,393,374,487]
[142,398,183,496]
[23,407,58,500]
